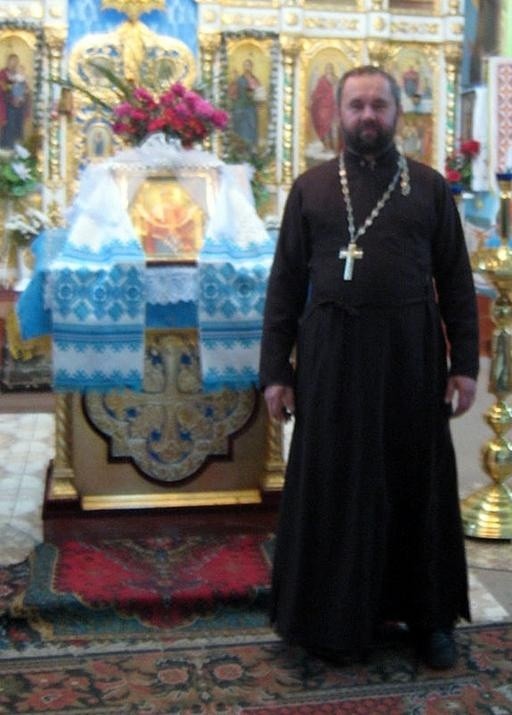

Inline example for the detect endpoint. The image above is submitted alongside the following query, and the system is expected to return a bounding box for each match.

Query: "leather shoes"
[409,622,456,671]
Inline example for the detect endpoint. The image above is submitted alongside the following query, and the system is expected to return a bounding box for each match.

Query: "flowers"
[444,140,481,194]
[41,58,233,147]
[1,133,44,199]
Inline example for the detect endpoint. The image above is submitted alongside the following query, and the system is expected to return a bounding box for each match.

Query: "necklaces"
[338,152,412,281]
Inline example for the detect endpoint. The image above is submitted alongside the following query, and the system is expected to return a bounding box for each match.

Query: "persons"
[396,66,432,163]
[5,65,27,107]
[258,67,479,670]
[0,54,33,148]
[306,63,340,152]
[228,59,261,151]
[115,6,152,88]
